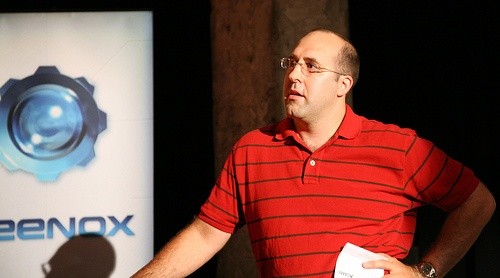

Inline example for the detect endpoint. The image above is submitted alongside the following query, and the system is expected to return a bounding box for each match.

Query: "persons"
[129,27,497,278]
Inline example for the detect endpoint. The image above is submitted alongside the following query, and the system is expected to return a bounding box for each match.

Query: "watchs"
[417,260,441,278]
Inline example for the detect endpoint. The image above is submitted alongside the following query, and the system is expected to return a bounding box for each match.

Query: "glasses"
[279,57,346,76]
